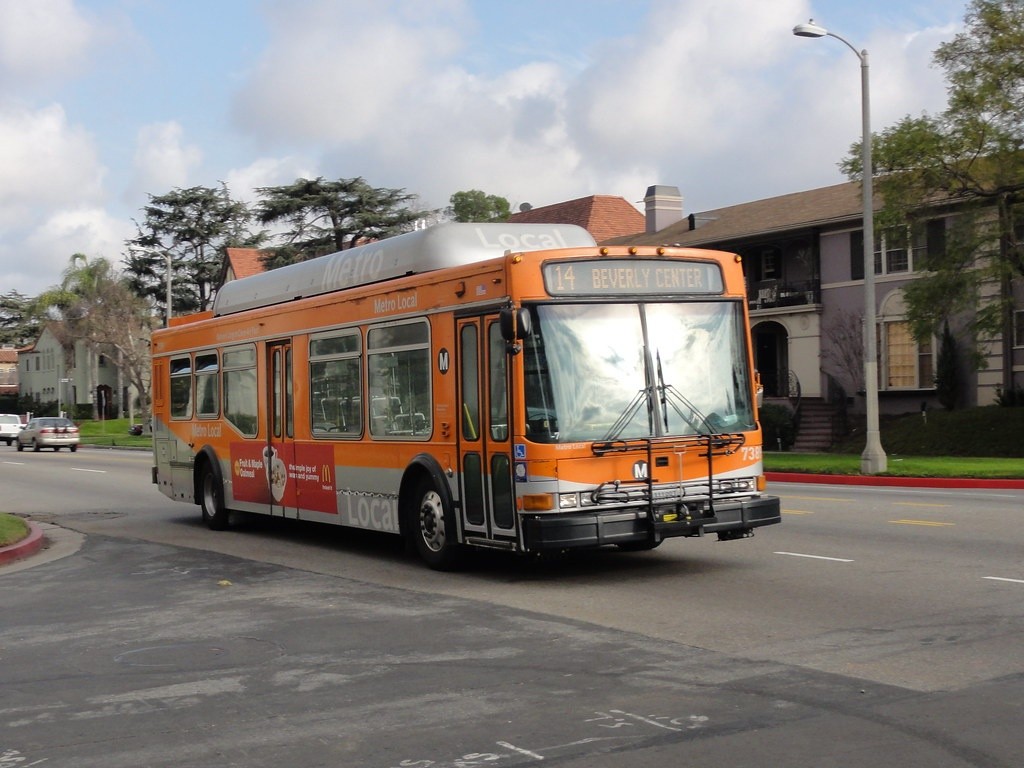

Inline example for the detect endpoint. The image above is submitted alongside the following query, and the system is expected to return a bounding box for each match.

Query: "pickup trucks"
[0,414,27,446]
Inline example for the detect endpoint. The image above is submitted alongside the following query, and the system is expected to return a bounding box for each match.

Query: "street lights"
[128,246,171,318]
[794,20,887,475]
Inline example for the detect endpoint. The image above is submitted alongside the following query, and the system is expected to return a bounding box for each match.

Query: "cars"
[127,417,152,436]
[16,417,80,452]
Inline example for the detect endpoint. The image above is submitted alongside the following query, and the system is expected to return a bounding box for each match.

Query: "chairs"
[311,375,426,437]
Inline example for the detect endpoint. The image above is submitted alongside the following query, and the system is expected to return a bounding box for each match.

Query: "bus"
[152,222,783,571]
